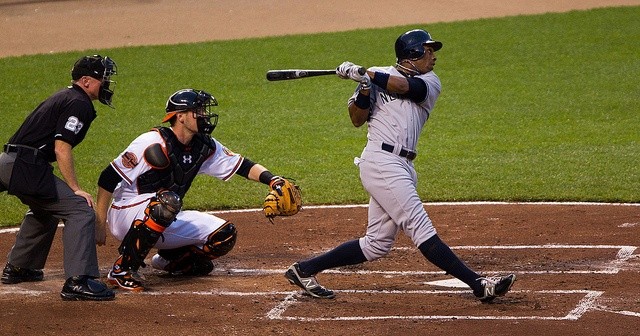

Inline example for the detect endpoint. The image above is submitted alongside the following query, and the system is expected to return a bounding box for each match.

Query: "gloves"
[349,65,372,90]
[336,60,356,78]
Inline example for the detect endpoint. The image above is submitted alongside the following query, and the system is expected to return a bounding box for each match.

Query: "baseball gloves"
[264,176,301,219]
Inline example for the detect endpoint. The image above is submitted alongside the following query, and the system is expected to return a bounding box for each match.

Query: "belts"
[3,143,17,153]
[382,142,416,159]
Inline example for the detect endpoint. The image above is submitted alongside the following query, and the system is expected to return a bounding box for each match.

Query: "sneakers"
[1,263,43,283]
[106,269,144,293]
[473,274,516,302]
[284,260,334,298]
[60,277,115,300]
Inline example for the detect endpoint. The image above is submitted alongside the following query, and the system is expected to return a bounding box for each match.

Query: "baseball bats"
[266,67,366,81]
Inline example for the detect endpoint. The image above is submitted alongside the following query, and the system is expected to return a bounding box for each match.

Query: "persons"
[0,54,117,301]
[284,28,517,304]
[96,89,303,293]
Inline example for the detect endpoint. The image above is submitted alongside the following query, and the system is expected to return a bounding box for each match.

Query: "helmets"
[162,88,218,148]
[394,27,442,62]
[72,53,117,108]
